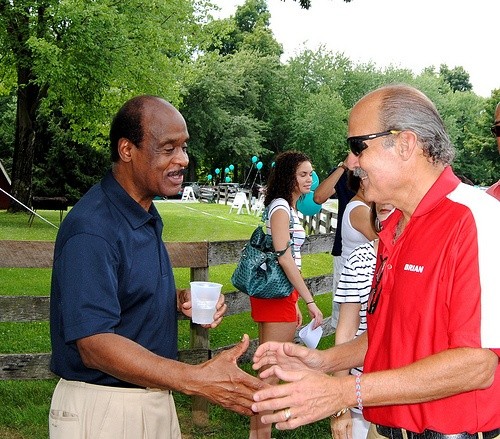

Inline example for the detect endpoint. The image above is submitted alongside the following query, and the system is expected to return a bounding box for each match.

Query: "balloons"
[215,168,220,174]
[207,174,212,180]
[224,168,229,173]
[272,162,276,167]
[225,176,231,182]
[229,165,234,170]
[251,156,257,163]
[256,162,263,171]
[296,170,323,218]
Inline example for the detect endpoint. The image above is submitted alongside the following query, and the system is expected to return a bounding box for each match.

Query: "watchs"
[338,161,349,172]
[328,408,349,419]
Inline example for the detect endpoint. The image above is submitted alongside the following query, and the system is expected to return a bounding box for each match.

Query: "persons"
[312,154,365,330]
[483,98,500,202]
[329,172,378,274]
[329,201,380,439]
[47,94,274,439]
[253,83,500,439]
[250,151,323,439]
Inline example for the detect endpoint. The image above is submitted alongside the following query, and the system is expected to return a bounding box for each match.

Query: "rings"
[284,407,291,420]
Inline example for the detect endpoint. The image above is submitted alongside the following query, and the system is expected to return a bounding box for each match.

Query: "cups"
[189,281,223,325]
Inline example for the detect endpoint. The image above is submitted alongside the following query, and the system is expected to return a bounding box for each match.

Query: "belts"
[88,375,147,389]
[376,424,500,439]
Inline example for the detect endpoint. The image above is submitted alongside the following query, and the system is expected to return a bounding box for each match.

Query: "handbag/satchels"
[231,205,296,298]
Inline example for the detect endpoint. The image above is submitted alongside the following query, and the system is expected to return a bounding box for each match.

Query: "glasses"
[491,125,500,138]
[347,129,402,157]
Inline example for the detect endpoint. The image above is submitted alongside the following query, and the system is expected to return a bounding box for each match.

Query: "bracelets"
[355,372,363,410]
[306,300,317,306]
